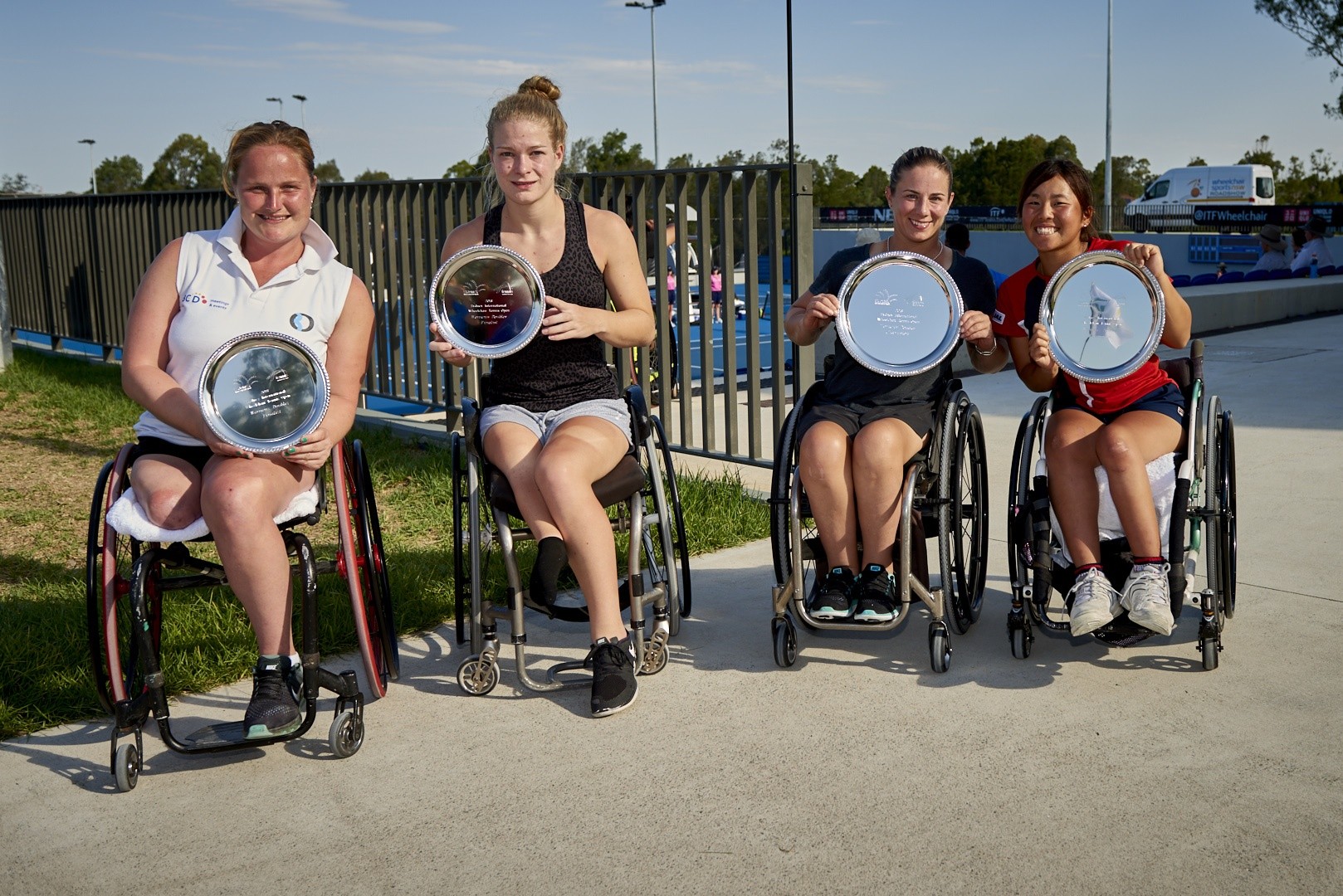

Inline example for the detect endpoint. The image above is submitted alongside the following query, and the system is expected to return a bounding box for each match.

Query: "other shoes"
[712,318,723,324]
[670,321,676,328]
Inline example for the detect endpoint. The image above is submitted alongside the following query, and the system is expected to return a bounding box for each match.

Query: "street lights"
[292,94,307,130]
[625,0,666,170]
[77,138,97,194]
[268,97,284,122]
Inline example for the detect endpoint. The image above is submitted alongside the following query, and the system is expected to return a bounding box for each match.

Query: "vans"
[1122,163,1276,234]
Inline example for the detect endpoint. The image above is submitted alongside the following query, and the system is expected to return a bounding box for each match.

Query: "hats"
[1296,215,1334,239]
[1254,224,1289,251]
[854,227,881,247]
[945,223,970,252]
[1216,262,1228,268]
[668,265,672,271]
[712,265,719,271]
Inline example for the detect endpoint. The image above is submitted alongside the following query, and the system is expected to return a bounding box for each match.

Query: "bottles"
[1309,254,1319,278]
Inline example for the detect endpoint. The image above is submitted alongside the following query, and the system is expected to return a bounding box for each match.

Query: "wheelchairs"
[766,356,989,673]
[84,432,399,791]
[450,383,693,699]
[1006,340,1240,664]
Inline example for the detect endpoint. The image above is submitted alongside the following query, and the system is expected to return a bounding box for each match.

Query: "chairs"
[1171,265,1343,288]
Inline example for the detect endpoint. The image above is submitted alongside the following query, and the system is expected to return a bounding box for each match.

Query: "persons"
[1216,262,1227,279]
[665,267,726,325]
[428,74,657,719]
[1249,201,1343,271]
[990,158,1193,637]
[784,146,1010,626]
[120,121,376,739]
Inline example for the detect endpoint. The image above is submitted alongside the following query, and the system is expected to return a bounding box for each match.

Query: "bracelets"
[975,338,997,356]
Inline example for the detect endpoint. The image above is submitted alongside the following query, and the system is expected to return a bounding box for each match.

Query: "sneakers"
[242,654,308,741]
[582,629,638,717]
[854,563,901,622]
[1061,566,1125,636]
[1117,562,1175,637]
[812,562,859,619]
